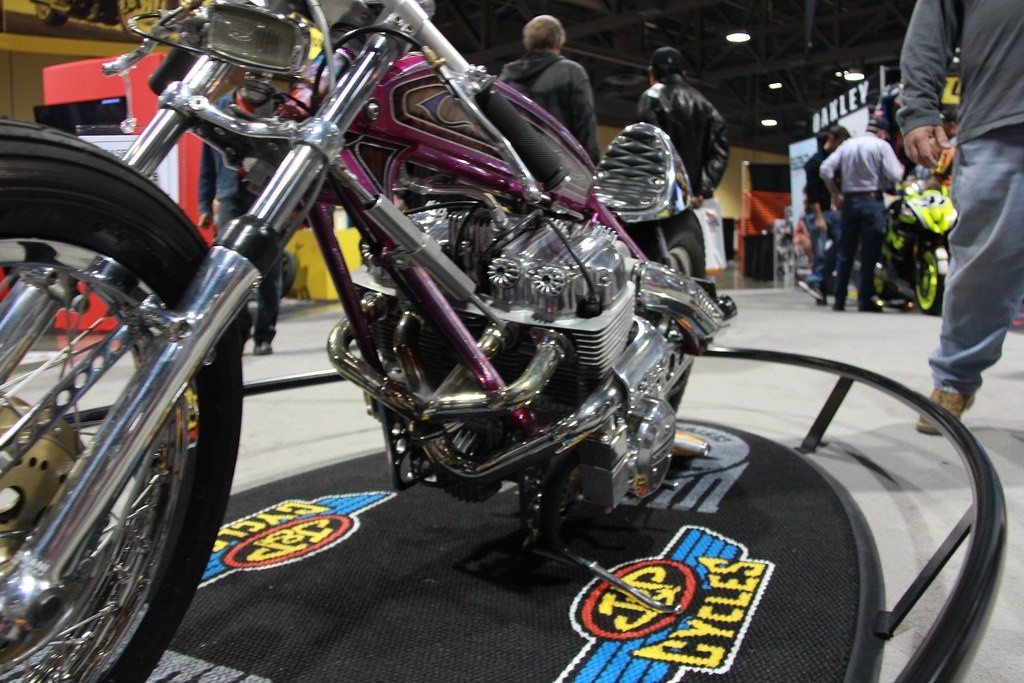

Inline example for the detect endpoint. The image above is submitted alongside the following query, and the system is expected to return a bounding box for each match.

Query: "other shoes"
[798,281,825,301]
[858,300,882,312]
[916,388,975,434]
[833,304,843,310]
[254,340,272,354]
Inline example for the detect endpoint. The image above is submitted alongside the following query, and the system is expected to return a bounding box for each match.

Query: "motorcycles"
[0,0,740,683]
[868,167,959,316]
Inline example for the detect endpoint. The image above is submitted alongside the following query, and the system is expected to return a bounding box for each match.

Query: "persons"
[897,0,1023,435]
[195,66,295,354]
[630,46,730,206]
[497,14,603,169]
[796,81,959,314]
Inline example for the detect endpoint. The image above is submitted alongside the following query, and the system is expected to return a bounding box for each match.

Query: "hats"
[868,116,892,141]
[650,47,686,79]
